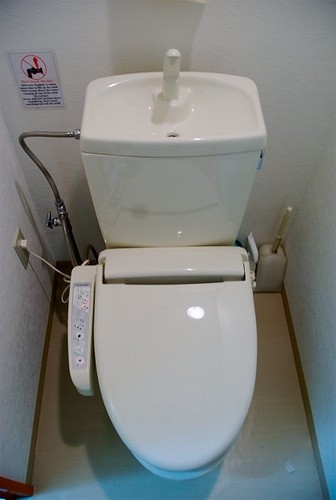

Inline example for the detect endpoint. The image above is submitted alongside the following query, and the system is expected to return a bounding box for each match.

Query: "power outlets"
[12,228,31,270]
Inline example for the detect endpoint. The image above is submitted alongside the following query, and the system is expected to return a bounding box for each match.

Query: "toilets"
[67,47,269,481]
